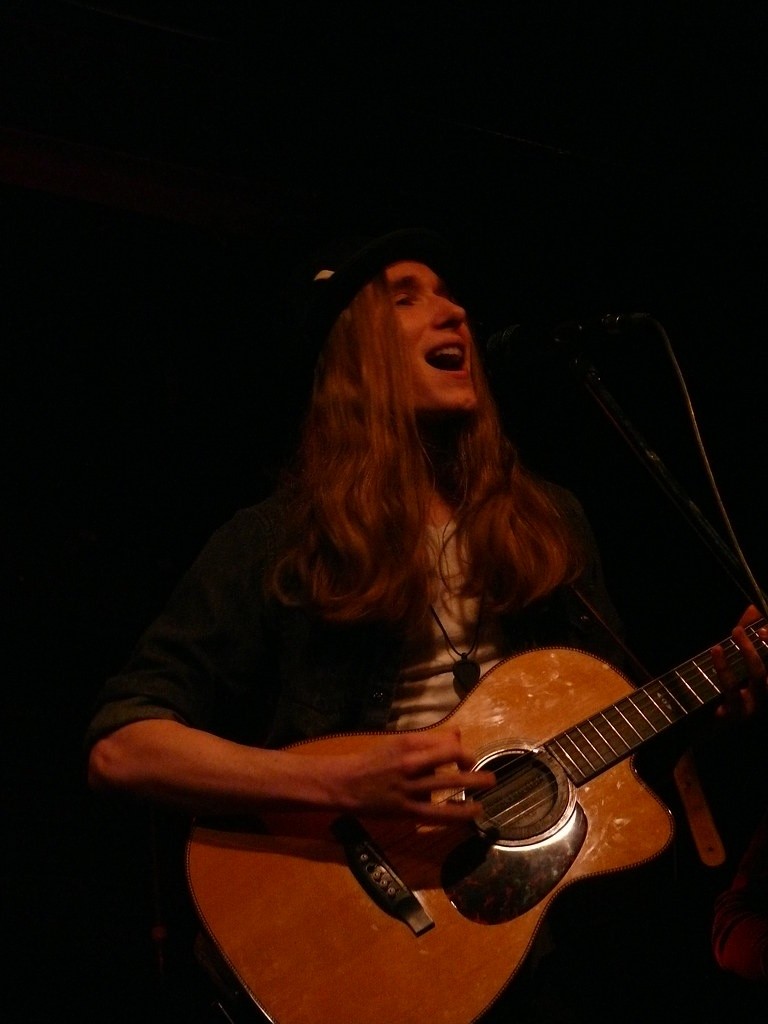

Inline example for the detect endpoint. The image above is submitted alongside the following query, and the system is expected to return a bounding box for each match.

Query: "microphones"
[486,313,650,368]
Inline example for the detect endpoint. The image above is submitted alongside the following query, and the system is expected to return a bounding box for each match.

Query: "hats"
[273,227,469,398]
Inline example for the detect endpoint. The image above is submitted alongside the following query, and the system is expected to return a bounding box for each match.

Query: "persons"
[88,226,768,1024]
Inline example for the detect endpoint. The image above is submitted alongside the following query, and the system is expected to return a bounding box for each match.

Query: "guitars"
[182,614,768,1023]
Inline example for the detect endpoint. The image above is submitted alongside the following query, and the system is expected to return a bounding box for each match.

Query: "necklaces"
[430,592,483,692]
